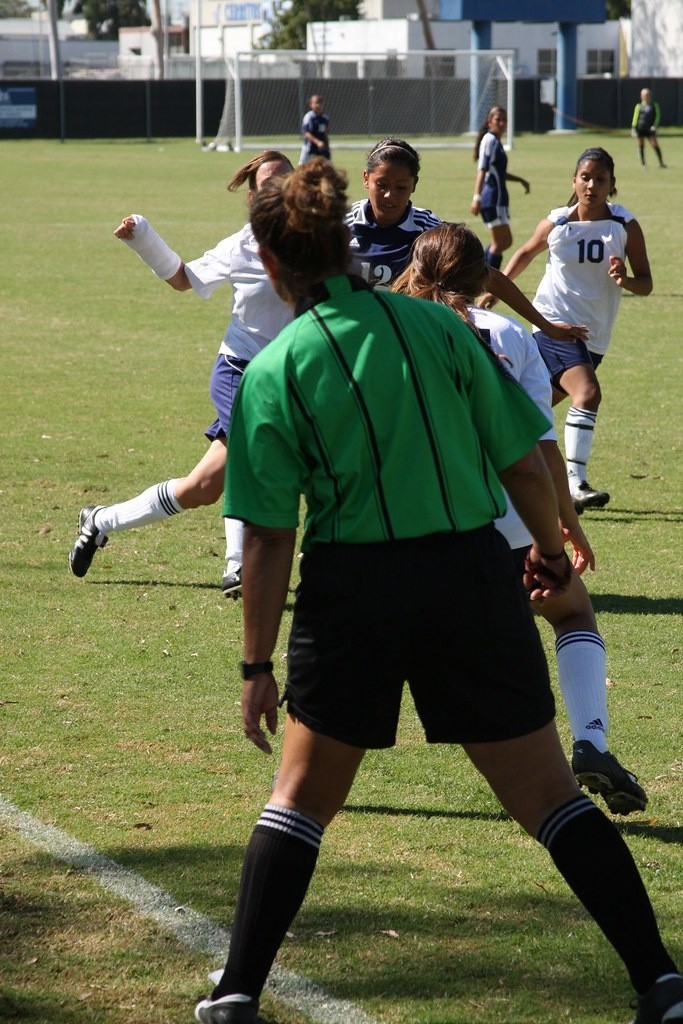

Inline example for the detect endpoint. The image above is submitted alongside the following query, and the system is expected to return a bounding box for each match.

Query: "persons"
[631,88,667,169]
[343,139,591,342]
[390,222,648,816]
[471,105,532,269]
[298,96,332,166]
[69,147,294,579]
[476,147,653,515]
[194,156,683,1024]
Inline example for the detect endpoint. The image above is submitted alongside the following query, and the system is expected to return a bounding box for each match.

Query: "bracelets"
[239,661,274,680]
[534,546,565,561]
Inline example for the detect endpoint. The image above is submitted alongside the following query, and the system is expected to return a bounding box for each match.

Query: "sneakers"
[572,740,648,816]
[629,971,683,1024]
[194,968,260,1024]
[69,506,108,577]
[222,566,242,595]
[573,480,610,516]
